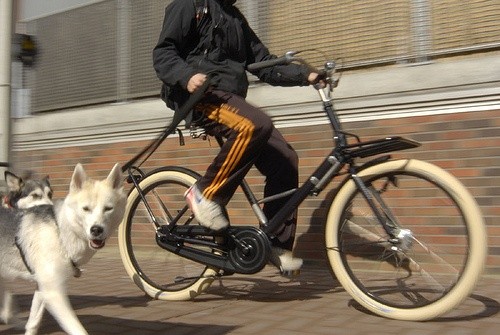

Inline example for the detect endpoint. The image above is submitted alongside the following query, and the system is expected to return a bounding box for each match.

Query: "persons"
[153,0,325,272]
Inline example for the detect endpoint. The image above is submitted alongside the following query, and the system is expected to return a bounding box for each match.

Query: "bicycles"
[118,48,487,321]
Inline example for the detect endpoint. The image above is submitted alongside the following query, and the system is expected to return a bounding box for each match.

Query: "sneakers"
[266,246,305,272]
[183,182,230,232]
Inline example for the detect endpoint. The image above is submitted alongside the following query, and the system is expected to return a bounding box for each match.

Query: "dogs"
[0,163,128,335]
[0,171,53,209]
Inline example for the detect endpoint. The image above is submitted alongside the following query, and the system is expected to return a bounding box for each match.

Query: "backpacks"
[159,0,223,128]
